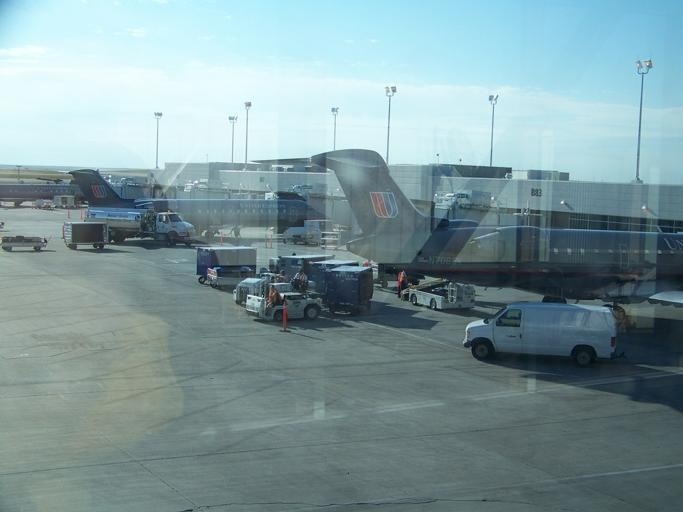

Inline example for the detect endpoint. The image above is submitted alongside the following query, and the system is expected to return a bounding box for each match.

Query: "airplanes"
[307,148,683,332]
[67,168,328,239]
[0,183,87,207]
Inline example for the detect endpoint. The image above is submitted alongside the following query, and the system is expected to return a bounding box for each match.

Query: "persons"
[265,284,282,314]
[275,269,286,284]
[299,272,309,299]
[291,273,299,291]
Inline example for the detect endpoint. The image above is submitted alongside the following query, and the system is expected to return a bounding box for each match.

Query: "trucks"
[462,302,620,365]
[84,210,195,246]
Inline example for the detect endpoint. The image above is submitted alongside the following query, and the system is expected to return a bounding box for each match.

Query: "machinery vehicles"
[283,217,340,248]
[398,279,476,313]
[231,272,323,324]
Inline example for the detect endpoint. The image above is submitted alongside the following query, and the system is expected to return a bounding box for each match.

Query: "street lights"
[154,111,162,169]
[330,106,340,151]
[228,115,238,163]
[487,94,499,166]
[384,85,397,165]
[15,165,22,180]
[244,101,251,163]
[633,58,653,184]
